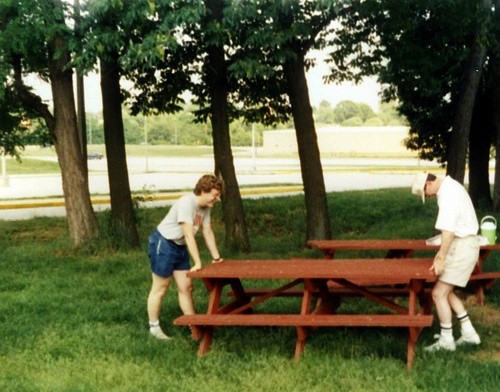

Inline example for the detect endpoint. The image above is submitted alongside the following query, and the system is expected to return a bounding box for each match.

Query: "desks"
[186,257,440,369]
[307,240,500,307]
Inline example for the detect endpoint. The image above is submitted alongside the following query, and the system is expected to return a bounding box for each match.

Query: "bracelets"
[214,257,220,262]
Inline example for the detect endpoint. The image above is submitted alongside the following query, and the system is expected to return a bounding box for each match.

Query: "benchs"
[227,288,434,314]
[173,314,433,342]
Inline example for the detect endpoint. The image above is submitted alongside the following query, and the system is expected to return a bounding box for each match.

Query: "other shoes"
[149,329,170,340]
[424,331,482,352]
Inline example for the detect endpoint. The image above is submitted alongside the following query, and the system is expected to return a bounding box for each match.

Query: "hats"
[412,172,428,205]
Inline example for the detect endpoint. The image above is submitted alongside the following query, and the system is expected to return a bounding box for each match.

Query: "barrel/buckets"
[480,215,497,247]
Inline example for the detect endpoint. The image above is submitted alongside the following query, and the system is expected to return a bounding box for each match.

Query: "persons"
[412,171,481,351]
[147,175,225,341]
